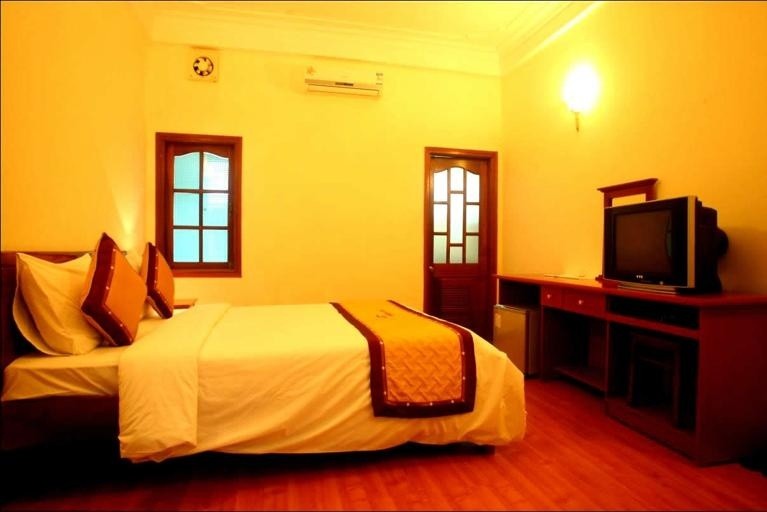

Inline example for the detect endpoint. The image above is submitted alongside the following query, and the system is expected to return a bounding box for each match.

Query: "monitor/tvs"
[602,196,728,295]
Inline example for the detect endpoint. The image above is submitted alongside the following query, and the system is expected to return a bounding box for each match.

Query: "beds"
[0,249,527,455]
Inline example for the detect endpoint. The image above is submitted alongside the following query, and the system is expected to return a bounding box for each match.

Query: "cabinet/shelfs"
[490,272,767,465]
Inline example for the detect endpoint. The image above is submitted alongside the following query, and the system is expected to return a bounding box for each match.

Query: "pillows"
[140,240,175,318]
[12,252,105,355]
[79,232,148,346]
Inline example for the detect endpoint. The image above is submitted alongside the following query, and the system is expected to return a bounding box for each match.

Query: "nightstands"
[173,297,197,309]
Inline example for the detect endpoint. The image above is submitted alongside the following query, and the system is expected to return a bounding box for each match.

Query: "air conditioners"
[306,65,387,98]
